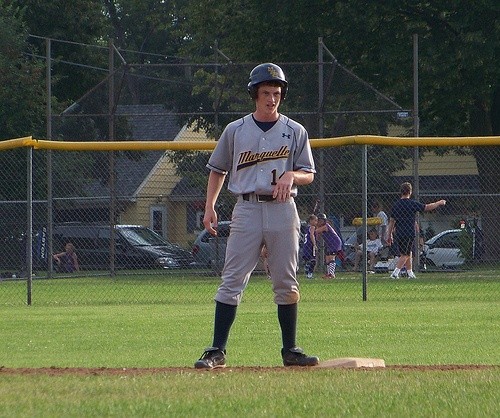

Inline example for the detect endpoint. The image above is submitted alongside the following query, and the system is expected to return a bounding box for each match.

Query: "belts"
[242,194,293,203]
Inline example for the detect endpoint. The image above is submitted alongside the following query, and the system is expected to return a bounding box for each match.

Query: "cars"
[342,215,386,268]
[419,227,476,270]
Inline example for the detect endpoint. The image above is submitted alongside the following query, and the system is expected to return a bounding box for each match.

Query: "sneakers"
[390,274,399,280]
[322,274,335,278]
[408,275,416,279]
[194,347,226,369]
[281,347,319,367]
[368,271,375,274]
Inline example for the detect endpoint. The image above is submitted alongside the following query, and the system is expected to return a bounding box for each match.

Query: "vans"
[35,221,196,272]
[191,220,354,272]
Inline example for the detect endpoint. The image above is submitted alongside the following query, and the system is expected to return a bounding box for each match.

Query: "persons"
[384,183,446,279]
[54,244,80,274]
[316,214,342,279]
[352,228,382,273]
[301,215,318,280]
[194,63,319,369]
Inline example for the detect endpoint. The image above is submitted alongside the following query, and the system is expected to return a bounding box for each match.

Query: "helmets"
[247,63,288,100]
[317,213,327,219]
